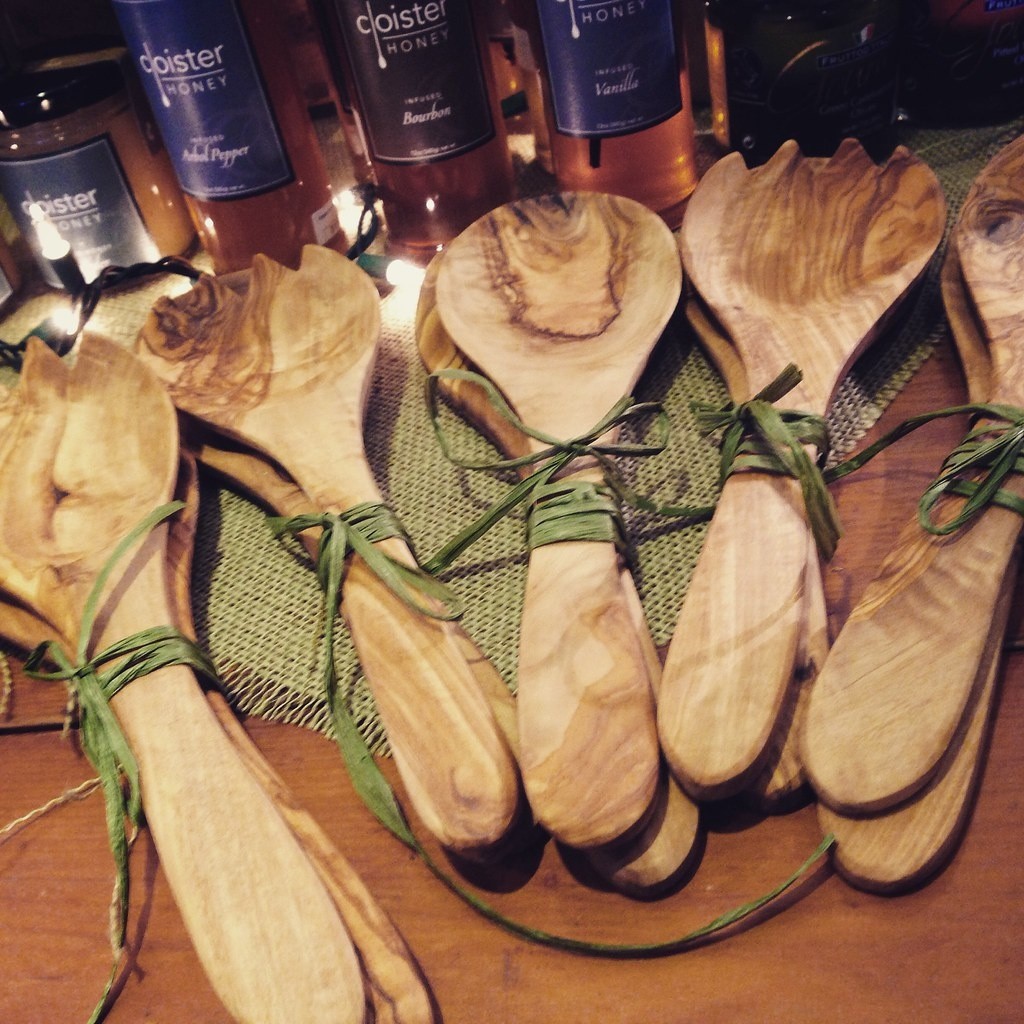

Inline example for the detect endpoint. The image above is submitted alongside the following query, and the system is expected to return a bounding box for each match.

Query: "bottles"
[1,3,941,292]
